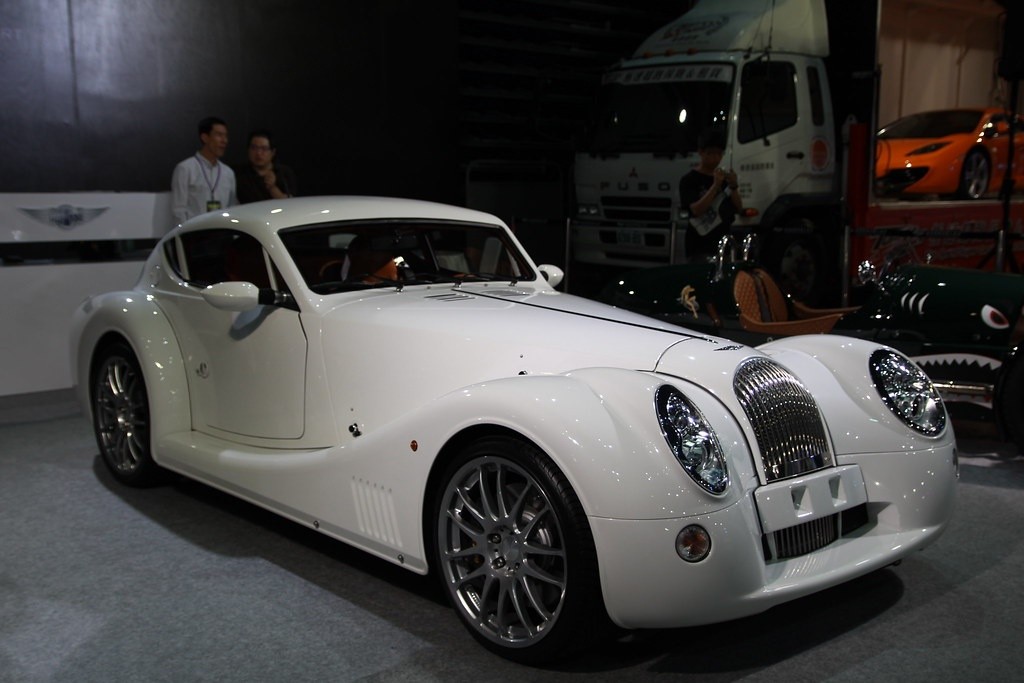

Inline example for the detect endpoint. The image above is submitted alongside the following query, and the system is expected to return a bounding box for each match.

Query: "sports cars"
[75,195,958,672]
[872,107,1023,201]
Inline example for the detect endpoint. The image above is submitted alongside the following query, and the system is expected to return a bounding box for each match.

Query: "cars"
[554,232,1023,455]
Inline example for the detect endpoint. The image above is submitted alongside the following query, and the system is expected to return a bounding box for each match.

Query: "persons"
[238,134,299,204]
[679,151,742,258]
[170,116,242,228]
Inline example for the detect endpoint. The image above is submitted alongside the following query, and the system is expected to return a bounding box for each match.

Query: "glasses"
[251,144,272,151]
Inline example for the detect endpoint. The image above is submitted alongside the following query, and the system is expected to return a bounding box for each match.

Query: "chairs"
[343,236,397,287]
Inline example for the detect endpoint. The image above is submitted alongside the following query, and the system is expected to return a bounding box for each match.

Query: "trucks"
[571,1,839,332]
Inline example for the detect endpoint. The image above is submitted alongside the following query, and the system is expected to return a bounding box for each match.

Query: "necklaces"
[200,149,217,166]
